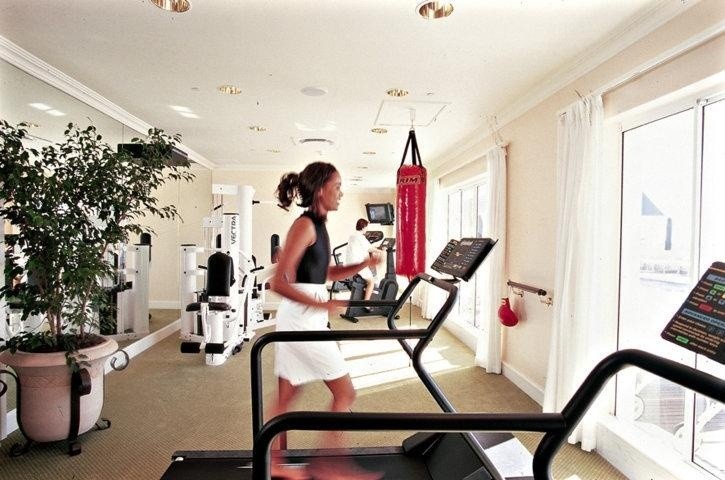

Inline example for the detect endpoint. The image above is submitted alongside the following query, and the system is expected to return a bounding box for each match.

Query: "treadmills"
[250,260,724,480]
[159,239,537,480]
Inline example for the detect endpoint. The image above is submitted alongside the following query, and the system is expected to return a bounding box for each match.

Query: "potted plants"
[2,116,193,457]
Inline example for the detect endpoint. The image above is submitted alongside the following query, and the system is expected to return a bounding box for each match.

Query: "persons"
[270,231,284,264]
[266,161,388,479]
[346,218,387,313]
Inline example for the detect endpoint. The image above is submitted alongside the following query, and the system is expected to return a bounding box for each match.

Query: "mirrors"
[0,35,214,444]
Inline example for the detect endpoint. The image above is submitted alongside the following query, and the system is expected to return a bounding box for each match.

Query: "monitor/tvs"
[365,203,395,224]
